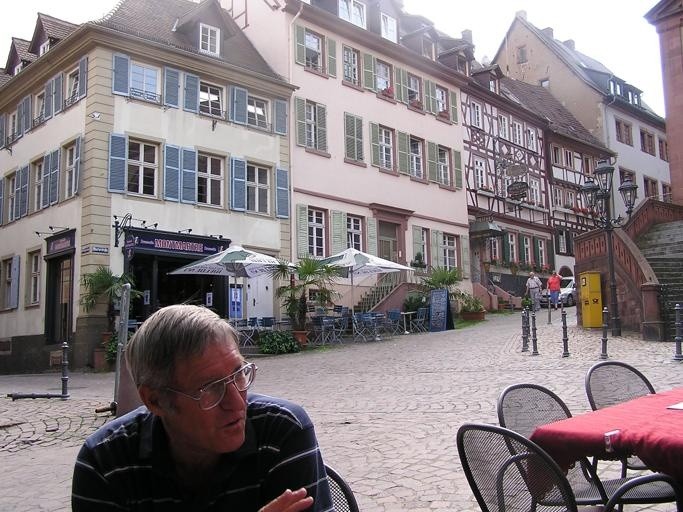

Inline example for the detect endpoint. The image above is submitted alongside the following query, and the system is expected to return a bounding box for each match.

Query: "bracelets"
[539,289,542,291]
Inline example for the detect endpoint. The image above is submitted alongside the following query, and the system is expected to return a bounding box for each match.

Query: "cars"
[539,276,576,308]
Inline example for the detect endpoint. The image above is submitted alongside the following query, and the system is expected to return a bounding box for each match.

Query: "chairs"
[223,301,430,355]
[455,358,682,511]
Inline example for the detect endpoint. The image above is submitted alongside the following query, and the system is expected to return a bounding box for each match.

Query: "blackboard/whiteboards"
[430,290,448,331]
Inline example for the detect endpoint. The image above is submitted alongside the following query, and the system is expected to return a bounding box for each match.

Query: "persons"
[524,271,543,311]
[547,270,563,312]
[69,303,338,512]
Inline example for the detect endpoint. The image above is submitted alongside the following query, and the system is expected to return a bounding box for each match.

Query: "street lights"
[582,160,638,337]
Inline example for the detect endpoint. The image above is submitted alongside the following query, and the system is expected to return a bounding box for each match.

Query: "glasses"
[164,360,258,412]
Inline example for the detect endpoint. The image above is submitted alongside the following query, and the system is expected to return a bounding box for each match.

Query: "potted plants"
[458,290,484,322]
[286,293,309,347]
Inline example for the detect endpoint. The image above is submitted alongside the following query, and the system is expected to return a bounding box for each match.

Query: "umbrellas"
[302,245,417,316]
[164,243,300,326]
